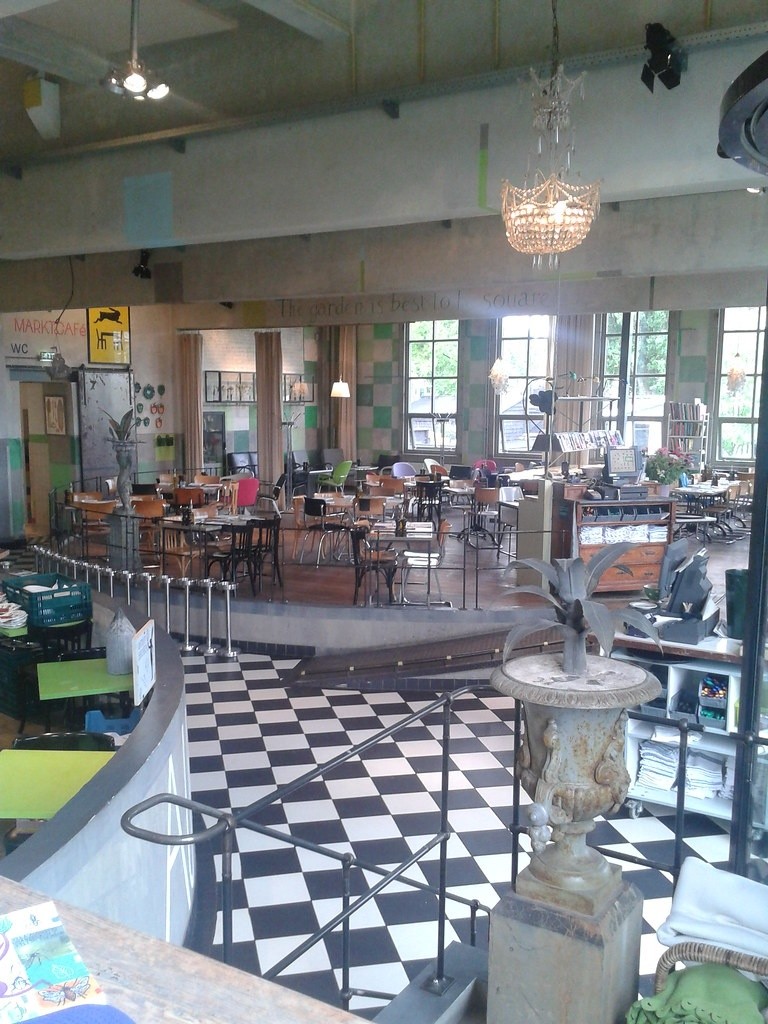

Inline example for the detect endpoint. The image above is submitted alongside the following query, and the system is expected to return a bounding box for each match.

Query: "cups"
[622,601,657,638]
[643,583,661,601]
[419,470,426,475]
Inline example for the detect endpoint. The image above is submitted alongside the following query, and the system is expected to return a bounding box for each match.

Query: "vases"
[657,485,669,498]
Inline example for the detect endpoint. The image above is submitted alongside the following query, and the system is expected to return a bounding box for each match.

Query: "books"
[668,397,707,471]
[635,745,736,805]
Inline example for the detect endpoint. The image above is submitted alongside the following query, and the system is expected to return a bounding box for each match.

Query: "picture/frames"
[86,306,132,365]
[285,374,301,403]
[301,374,314,402]
[220,371,241,403]
[240,372,255,403]
[43,393,68,437]
[282,374,286,403]
[254,372,257,403]
[205,371,221,402]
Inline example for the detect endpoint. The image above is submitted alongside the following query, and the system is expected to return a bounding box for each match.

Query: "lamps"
[489,354,510,396]
[501,0,603,271]
[330,375,351,398]
[726,343,747,397]
[98,0,171,102]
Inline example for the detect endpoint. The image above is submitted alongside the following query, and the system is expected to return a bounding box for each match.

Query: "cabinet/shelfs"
[667,413,710,473]
[550,482,678,598]
[586,615,768,843]
[516,477,553,589]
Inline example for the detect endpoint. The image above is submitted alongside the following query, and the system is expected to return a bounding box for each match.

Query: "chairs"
[292,450,311,467]
[66,457,754,610]
[45,647,131,733]
[85,703,141,750]
[321,448,355,492]
[250,451,259,475]
[13,732,116,751]
[16,621,93,734]
[227,452,252,475]
[36,659,133,733]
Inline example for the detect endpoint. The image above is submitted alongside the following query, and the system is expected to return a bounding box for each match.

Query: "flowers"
[644,446,694,486]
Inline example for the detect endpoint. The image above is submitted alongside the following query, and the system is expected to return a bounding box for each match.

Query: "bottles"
[168,467,186,489]
[188,498,194,524]
[65,482,74,503]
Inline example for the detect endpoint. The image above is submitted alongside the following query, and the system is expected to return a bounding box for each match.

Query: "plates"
[0,585,85,629]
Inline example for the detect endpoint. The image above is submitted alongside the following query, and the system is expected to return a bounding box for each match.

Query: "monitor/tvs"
[658,537,714,619]
[608,446,639,478]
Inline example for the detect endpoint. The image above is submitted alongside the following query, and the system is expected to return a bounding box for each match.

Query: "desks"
[0,748,116,819]
[364,480,416,496]
[491,465,582,483]
[159,515,266,578]
[495,500,519,559]
[698,480,747,528]
[65,498,143,560]
[443,487,495,541]
[675,487,721,544]
[158,482,223,504]
[367,522,434,610]
[687,484,728,538]
[350,466,379,491]
[0,875,375,1024]
[313,496,403,562]
[0,620,85,638]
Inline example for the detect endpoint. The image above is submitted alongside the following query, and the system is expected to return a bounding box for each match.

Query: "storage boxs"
[647,664,667,698]
[639,702,667,719]
[698,678,727,710]
[669,688,698,724]
[697,705,726,731]
[0,640,65,721]
[2,572,93,628]
